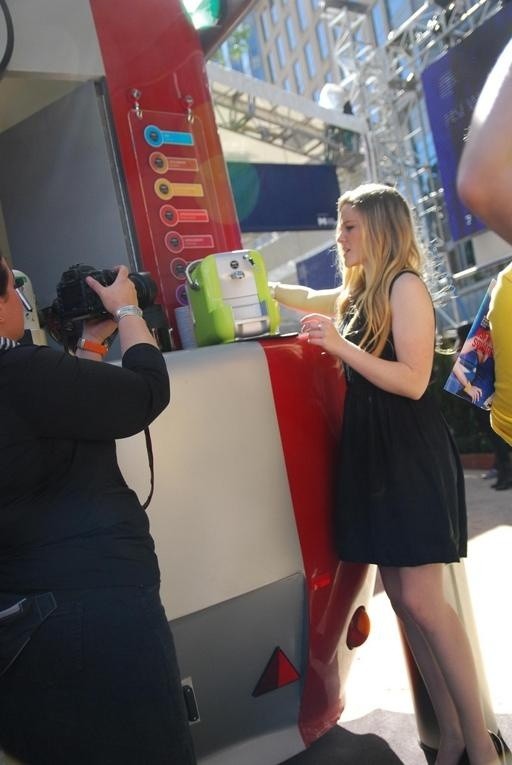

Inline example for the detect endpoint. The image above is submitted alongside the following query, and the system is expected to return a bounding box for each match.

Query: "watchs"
[113,305,143,323]
[76,338,108,357]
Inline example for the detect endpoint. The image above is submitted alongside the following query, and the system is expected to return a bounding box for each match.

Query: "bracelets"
[268,281,280,299]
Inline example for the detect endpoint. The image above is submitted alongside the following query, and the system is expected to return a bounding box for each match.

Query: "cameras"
[37,262,157,326]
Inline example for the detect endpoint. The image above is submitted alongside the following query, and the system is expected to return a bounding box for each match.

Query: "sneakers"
[481,468,497,479]
[491,478,512,490]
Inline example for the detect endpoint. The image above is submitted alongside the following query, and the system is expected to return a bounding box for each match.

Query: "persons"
[268,181,512,765]
[455,35,512,248]
[489,261,512,449]
[452,331,496,410]
[0,254,196,765]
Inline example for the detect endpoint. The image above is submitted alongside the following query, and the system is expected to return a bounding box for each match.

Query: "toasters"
[183,247,281,346]
